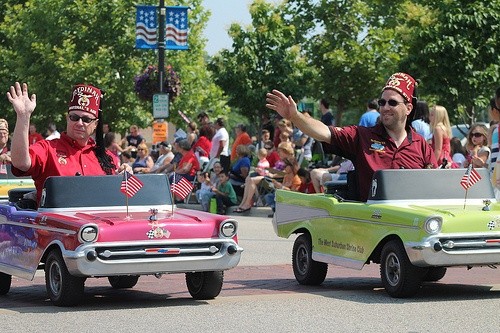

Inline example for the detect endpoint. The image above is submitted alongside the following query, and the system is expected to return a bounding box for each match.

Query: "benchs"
[323,180,347,202]
[8,188,36,208]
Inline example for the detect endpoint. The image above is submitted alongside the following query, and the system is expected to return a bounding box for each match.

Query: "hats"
[0,119,9,132]
[67,84,101,118]
[381,72,416,102]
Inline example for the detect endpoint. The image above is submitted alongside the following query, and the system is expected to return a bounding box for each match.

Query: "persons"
[0,71,500,218]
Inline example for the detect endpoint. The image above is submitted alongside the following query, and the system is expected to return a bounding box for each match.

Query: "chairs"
[183,158,283,206]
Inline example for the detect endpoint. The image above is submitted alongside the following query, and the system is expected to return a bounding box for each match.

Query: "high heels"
[232,206,251,216]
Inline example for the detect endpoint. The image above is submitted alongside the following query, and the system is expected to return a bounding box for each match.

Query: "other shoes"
[268,214,273,217]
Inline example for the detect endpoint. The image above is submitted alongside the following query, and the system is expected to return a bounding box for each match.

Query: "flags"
[169,172,193,198]
[461,161,482,190]
[120,169,144,197]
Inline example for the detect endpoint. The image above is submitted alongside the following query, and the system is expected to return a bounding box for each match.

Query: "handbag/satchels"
[210,194,226,213]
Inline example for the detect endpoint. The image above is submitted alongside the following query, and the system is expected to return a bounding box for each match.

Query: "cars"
[0,176,244,307]
[271,168,500,298]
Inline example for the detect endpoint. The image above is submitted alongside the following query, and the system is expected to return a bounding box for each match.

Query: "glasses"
[378,99,407,106]
[68,113,96,123]
[470,132,484,138]
